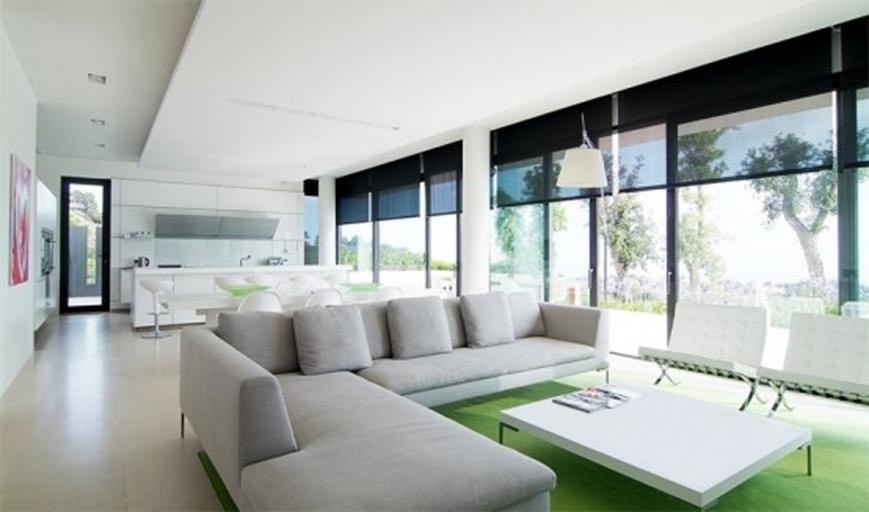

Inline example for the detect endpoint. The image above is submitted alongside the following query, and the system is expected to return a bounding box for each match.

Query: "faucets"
[239,255,252,266]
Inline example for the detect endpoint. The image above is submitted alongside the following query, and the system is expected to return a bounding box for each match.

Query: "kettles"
[138,256,149,267]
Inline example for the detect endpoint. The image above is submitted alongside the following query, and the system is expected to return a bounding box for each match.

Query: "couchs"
[180,287,609,510]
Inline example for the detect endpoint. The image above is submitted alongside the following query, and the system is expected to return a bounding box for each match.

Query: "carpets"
[197,378,868,512]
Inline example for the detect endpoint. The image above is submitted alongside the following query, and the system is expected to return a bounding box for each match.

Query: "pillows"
[293,306,372,375]
[458,290,515,349]
[387,295,452,359]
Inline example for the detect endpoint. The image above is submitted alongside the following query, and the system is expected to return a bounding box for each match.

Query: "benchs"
[754,311,869,423]
[638,302,769,410]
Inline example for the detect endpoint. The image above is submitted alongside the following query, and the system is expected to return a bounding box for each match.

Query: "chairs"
[139,270,407,340]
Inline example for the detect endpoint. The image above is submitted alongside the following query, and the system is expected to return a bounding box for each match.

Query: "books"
[552,380,641,413]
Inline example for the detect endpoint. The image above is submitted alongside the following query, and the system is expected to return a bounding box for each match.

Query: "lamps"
[555,113,609,306]
